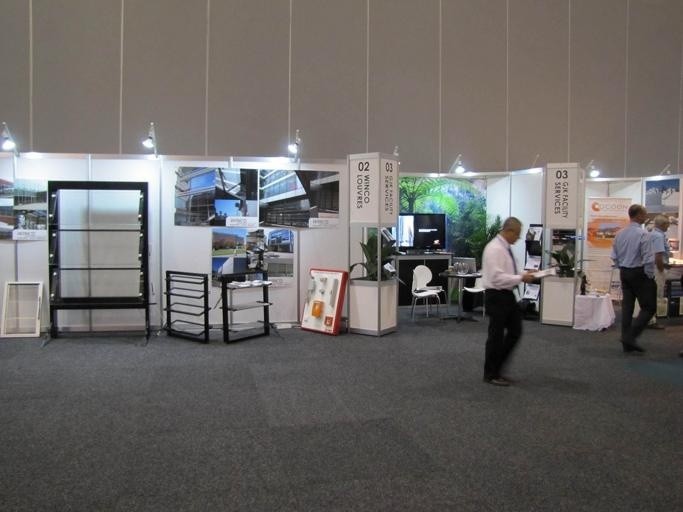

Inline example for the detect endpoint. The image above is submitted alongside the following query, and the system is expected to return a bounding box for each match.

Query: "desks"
[573,293,616,332]
[438,272,482,323]
[665,258,683,318]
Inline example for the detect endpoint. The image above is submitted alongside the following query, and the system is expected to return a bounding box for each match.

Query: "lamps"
[449,153,465,175]
[0,122,20,155]
[659,162,672,175]
[586,160,600,178]
[287,130,303,163]
[142,122,159,157]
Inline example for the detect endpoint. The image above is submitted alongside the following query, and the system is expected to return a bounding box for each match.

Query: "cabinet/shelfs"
[522,224,543,322]
[398,255,451,308]
[164,268,272,344]
[41,181,157,348]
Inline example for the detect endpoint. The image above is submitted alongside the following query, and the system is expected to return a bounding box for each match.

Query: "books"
[531,267,555,279]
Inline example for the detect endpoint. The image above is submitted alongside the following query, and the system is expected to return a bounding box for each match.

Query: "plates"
[424,252,433,255]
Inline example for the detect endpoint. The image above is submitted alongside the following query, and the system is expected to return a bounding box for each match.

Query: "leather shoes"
[483,370,509,386]
[619,334,647,353]
[646,324,666,330]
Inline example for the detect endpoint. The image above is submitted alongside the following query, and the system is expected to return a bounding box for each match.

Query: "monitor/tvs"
[390,212,446,254]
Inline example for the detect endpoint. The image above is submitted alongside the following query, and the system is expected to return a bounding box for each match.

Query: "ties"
[508,248,520,296]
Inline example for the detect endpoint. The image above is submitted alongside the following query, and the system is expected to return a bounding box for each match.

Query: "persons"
[632,216,671,329]
[482,217,540,387]
[610,204,657,353]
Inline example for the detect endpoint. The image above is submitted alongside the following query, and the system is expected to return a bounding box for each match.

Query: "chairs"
[459,277,486,320]
[416,265,449,317]
[411,270,441,322]
